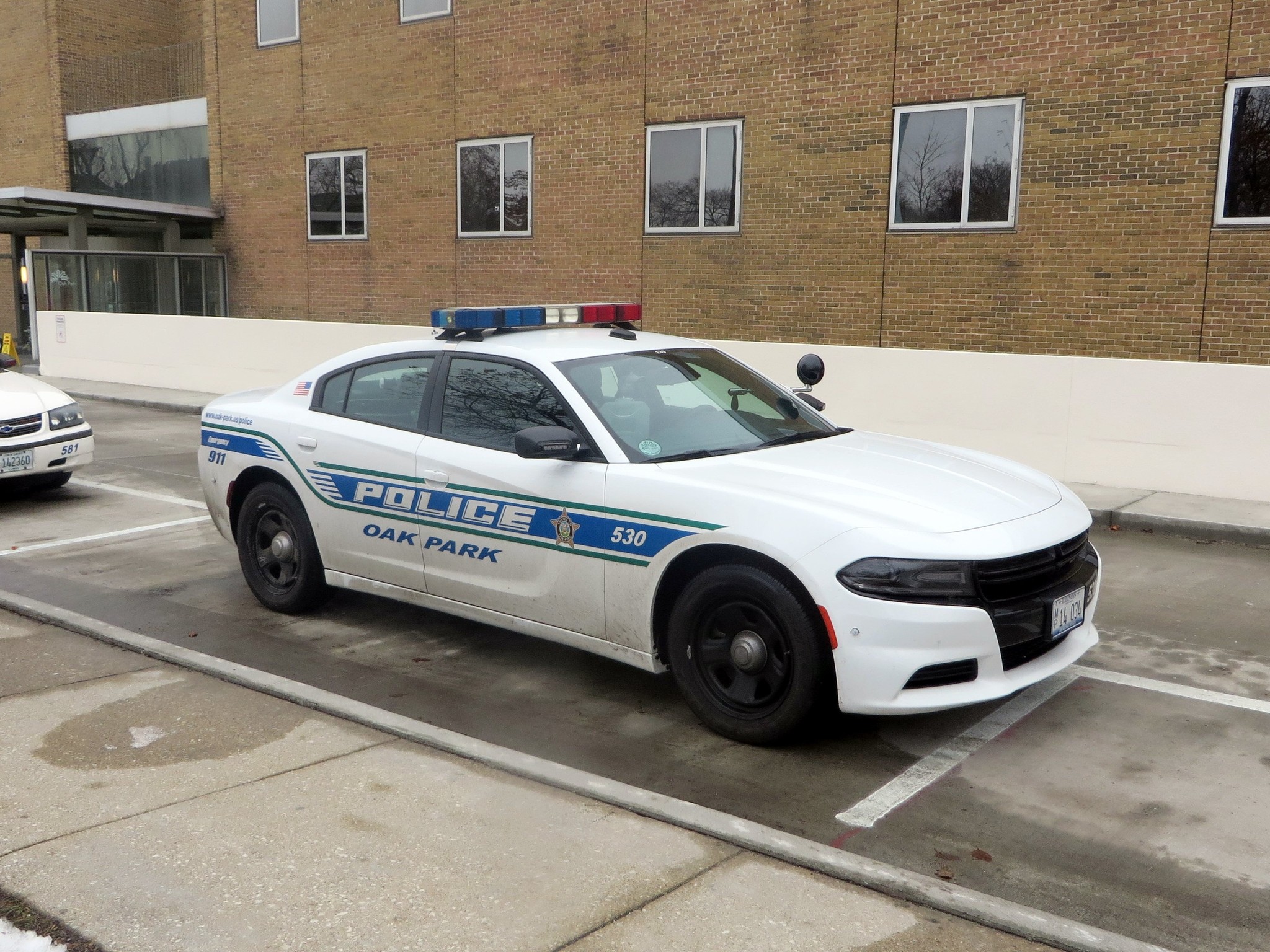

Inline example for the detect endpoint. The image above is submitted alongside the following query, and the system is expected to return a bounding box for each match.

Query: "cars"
[0,351,94,490]
[198,301,1101,750]
